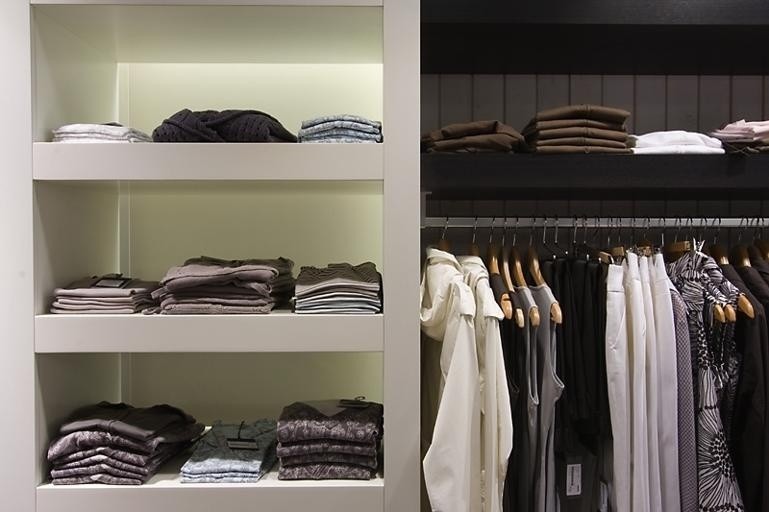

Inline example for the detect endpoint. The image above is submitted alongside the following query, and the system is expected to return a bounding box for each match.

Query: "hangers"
[428,211,768,328]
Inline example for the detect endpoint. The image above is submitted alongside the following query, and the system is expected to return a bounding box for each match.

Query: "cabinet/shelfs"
[33,351,386,488]
[32,178,384,318]
[418,1,768,510]
[28,3,385,146]
